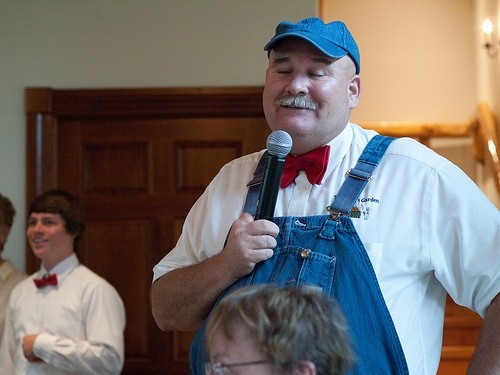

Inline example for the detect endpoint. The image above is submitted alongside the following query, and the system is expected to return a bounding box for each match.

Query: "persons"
[202,284,358,375]
[152,17,500,375]
[0,194,32,340]
[0,187,126,375]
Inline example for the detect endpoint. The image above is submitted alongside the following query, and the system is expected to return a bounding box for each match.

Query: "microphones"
[255,130,293,223]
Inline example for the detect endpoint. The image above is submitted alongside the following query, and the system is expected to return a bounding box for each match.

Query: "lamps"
[482,16,500,59]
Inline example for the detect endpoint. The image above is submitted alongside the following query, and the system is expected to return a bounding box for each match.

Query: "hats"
[263,17,362,73]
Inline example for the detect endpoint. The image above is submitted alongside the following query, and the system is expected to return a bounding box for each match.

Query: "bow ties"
[279,145,330,188]
[34,274,58,288]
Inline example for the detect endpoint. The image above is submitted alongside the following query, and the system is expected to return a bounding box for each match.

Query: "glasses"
[203,358,274,375]
